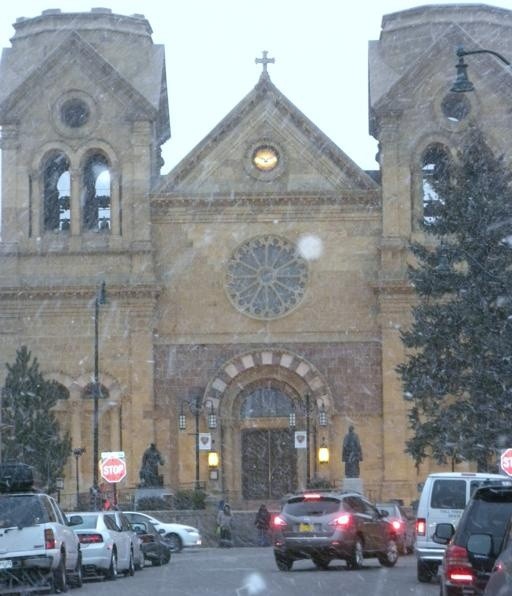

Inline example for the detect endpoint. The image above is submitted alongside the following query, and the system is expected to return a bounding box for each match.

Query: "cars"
[122,511,201,553]
[63,511,169,579]
[374,504,414,555]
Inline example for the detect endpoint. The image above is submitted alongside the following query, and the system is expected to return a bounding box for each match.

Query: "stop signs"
[501,448,512,476]
[100,457,127,484]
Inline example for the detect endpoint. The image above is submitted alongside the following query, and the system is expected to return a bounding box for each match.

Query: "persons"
[140,442,164,476]
[216,503,234,549]
[341,425,363,478]
[254,504,270,546]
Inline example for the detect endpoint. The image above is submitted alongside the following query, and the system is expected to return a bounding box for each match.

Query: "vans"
[414,472,511,583]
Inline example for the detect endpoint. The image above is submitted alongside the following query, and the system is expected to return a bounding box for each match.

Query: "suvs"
[0,494,83,591]
[440,487,511,596]
[271,493,399,571]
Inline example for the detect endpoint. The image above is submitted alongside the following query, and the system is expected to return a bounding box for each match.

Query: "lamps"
[208,451,218,468]
[319,443,329,463]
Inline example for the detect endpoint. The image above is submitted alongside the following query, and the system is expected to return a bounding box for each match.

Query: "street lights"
[291,392,328,490]
[451,48,512,92]
[179,396,216,488]
[94,281,112,508]
